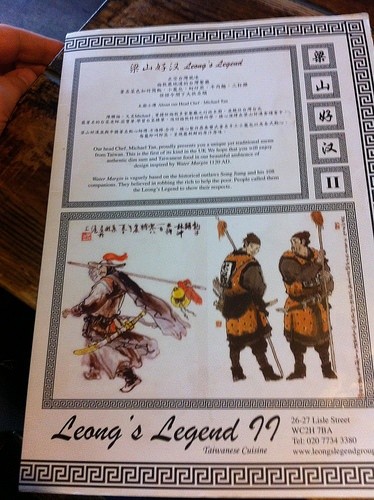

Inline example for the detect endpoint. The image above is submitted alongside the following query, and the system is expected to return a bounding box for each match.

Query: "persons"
[2,20,69,139]
